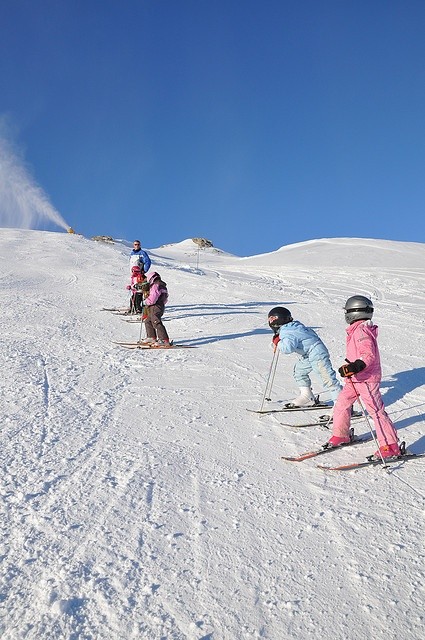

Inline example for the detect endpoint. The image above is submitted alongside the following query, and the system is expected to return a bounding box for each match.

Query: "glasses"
[134,244,140,245]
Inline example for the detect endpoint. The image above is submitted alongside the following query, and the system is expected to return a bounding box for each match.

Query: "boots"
[328,400,353,417]
[290,387,315,406]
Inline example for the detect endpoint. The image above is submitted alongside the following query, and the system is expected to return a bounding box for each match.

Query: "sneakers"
[152,340,169,346]
[375,444,401,458]
[142,337,156,343]
[328,436,350,446]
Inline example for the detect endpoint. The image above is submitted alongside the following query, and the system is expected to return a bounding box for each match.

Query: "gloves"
[140,300,146,308]
[272,334,280,345]
[339,358,366,377]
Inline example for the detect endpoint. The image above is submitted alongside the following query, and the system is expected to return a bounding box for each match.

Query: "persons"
[129,240,151,273]
[133,275,149,315]
[127,266,142,313]
[268,306,343,409]
[322,295,402,460]
[141,271,170,347]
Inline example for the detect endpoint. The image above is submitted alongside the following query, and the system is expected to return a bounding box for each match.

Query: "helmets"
[344,295,374,324]
[268,307,293,332]
[132,266,141,276]
[148,272,161,284]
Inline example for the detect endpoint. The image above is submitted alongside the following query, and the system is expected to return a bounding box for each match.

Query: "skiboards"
[112,313,141,316]
[100,308,134,311]
[281,428,425,470]
[121,318,171,323]
[112,342,199,349]
[247,404,370,427]
